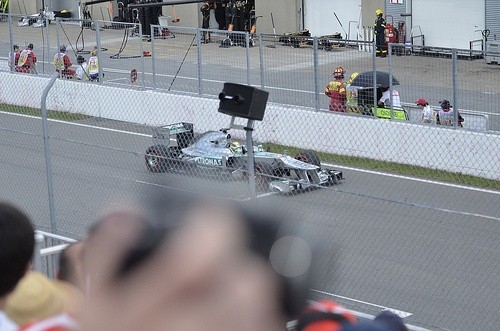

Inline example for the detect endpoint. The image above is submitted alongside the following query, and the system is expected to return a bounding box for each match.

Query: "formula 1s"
[144,121,345,197]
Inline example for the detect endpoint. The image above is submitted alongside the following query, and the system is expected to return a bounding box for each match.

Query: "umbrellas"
[350,71,400,87]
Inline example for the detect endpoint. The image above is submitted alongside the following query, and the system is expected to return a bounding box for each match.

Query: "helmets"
[417,97,425,104]
[376,10,383,15]
[60,45,65,51]
[349,72,358,82]
[334,67,344,76]
[441,100,450,109]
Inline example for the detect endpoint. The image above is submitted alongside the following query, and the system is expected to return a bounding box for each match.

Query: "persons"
[0,193,411,331]
[18,43,37,73]
[325,66,348,112]
[72,55,91,81]
[230,142,244,153]
[200,4,212,40]
[436,99,464,128]
[345,73,363,106]
[8,45,21,71]
[54,45,76,75]
[374,9,388,58]
[88,50,104,82]
[378,87,403,110]
[414,98,435,124]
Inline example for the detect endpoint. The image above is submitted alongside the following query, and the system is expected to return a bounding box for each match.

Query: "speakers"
[217,82,269,121]
